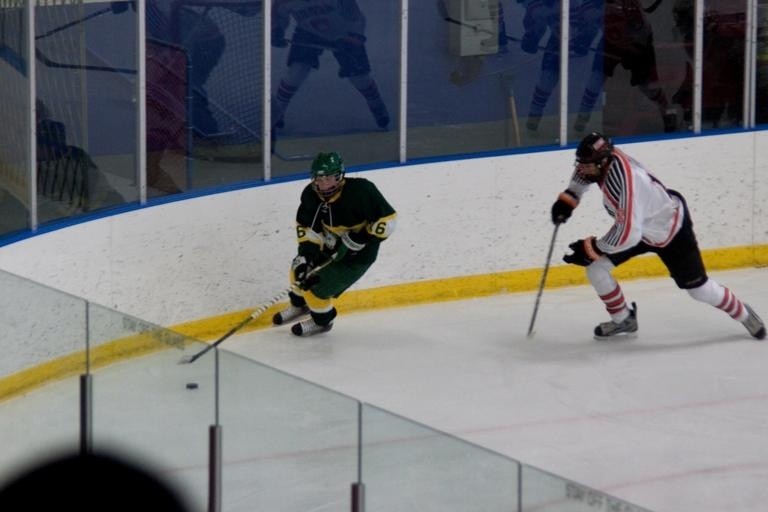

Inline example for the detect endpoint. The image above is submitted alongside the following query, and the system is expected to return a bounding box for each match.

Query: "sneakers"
[274,109,284,128]
[594,310,637,335]
[273,303,309,324]
[528,113,541,129]
[575,115,590,130]
[742,303,764,335]
[292,318,333,334]
[369,100,389,126]
[662,108,676,132]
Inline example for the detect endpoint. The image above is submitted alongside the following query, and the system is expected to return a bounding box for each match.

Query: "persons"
[573,1,678,137]
[272,150,396,337]
[518,1,613,135]
[146,1,263,145]
[267,0,392,139]
[551,131,766,342]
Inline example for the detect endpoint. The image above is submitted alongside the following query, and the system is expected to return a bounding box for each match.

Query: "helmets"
[575,133,612,183]
[311,152,344,197]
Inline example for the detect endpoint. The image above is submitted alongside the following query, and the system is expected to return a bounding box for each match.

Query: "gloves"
[293,255,319,290]
[271,34,288,47]
[332,231,366,263]
[552,190,579,223]
[521,32,540,53]
[336,34,366,78]
[563,237,604,266]
[570,34,590,57]
